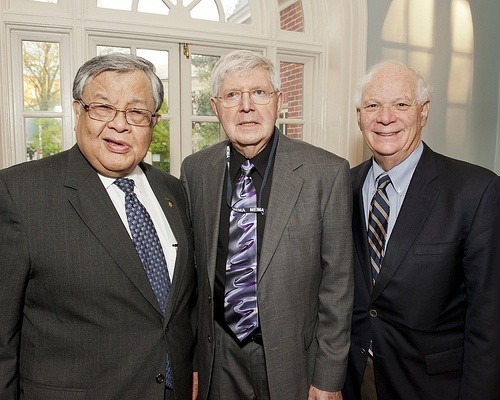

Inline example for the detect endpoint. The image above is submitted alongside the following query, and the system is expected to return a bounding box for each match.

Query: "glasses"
[211,90,277,107]
[81,97,157,126]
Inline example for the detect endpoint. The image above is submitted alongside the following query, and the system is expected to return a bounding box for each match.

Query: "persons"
[178,51,355,400]
[1,52,194,400]
[343,59,500,400]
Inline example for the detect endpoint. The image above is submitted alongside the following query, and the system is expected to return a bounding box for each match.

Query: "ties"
[222,160,260,342]
[115,178,170,316]
[367,174,392,356]
